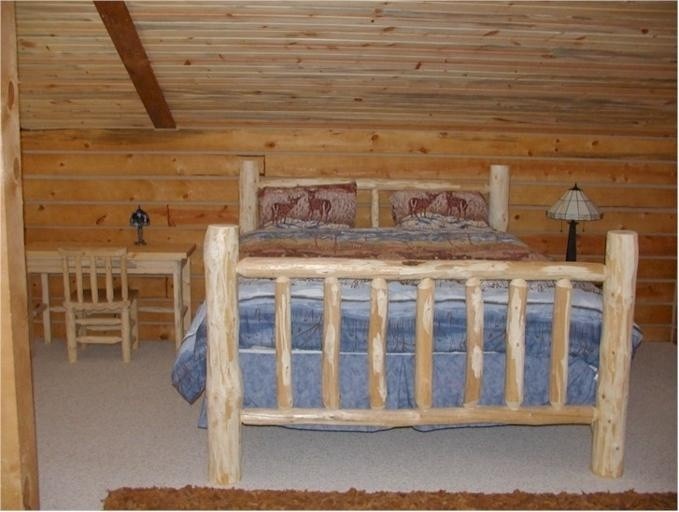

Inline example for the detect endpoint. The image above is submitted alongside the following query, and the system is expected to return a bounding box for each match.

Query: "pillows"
[258,181,358,229]
[388,190,488,230]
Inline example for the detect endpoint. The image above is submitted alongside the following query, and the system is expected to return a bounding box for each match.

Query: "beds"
[171,158,644,485]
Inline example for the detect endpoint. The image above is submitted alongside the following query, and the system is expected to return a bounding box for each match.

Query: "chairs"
[56,246,139,362]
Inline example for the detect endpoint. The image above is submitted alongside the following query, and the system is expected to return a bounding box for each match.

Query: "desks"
[25,242,197,351]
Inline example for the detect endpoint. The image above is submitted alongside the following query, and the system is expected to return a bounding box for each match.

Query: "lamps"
[547,181,601,262]
[130,204,151,245]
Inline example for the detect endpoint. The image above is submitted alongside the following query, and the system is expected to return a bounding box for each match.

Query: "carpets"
[103,488,678,510]
